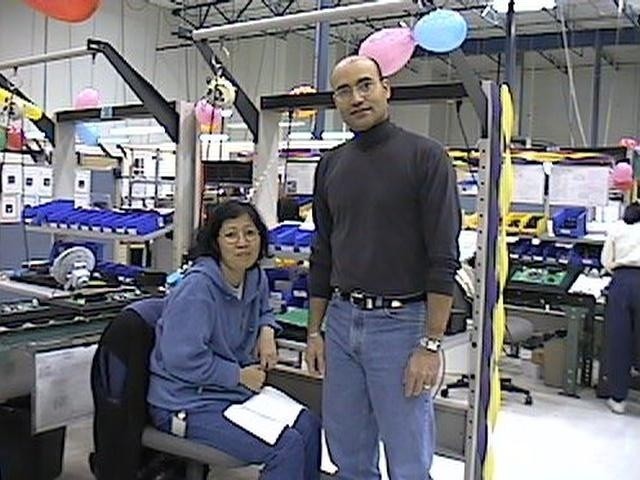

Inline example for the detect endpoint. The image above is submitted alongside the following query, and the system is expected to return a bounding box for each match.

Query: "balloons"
[359,26,414,77]
[613,160,633,183]
[24,1,101,23]
[415,8,468,52]
[75,88,100,108]
[290,84,319,116]
[195,100,222,126]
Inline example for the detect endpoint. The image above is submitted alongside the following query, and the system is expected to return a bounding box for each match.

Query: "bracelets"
[419,336,441,353]
[309,331,319,338]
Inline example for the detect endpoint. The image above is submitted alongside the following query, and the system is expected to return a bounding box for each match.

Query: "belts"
[332,287,427,313]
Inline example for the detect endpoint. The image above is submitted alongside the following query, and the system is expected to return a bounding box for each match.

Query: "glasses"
[217,228,258,245]
[332,80,376,104]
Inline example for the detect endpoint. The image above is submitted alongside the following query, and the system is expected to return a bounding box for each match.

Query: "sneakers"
[606,397,628,415]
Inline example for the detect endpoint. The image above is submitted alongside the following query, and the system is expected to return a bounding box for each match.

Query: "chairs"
[441,263,535,407]
[89,297,256,478]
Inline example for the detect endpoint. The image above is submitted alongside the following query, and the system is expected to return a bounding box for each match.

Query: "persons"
[303,54,462,480]
[149,199,323,479]
[596,200,640,415]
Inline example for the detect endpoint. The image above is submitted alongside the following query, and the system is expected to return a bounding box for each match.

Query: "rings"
[423,383,432,390]
